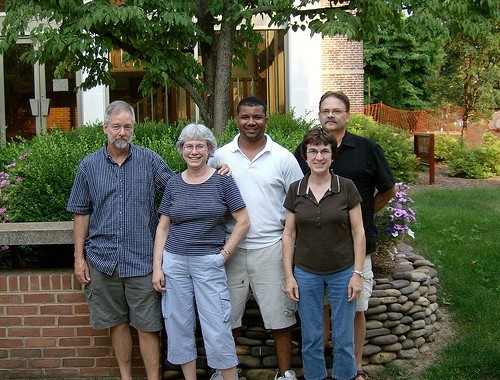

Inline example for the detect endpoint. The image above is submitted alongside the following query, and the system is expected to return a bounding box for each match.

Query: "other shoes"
[274,369,297,380]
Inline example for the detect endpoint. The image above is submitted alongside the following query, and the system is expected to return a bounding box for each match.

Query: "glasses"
[320,108,347,116]
[306,148,332,156]
[183,143,208,152]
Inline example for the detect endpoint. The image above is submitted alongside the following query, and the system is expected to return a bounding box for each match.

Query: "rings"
[152,286,154,288]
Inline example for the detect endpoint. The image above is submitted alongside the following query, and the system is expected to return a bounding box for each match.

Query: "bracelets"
[221,246,231,257]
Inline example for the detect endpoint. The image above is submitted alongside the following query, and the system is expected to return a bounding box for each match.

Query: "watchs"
[352,270,364,277]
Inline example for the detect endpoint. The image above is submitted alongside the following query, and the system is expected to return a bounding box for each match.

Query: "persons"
[152,123,252,380]
[280,126,366,380]
[66,99,232,380]
[206,95,305,380]
[292,90,396,380]
